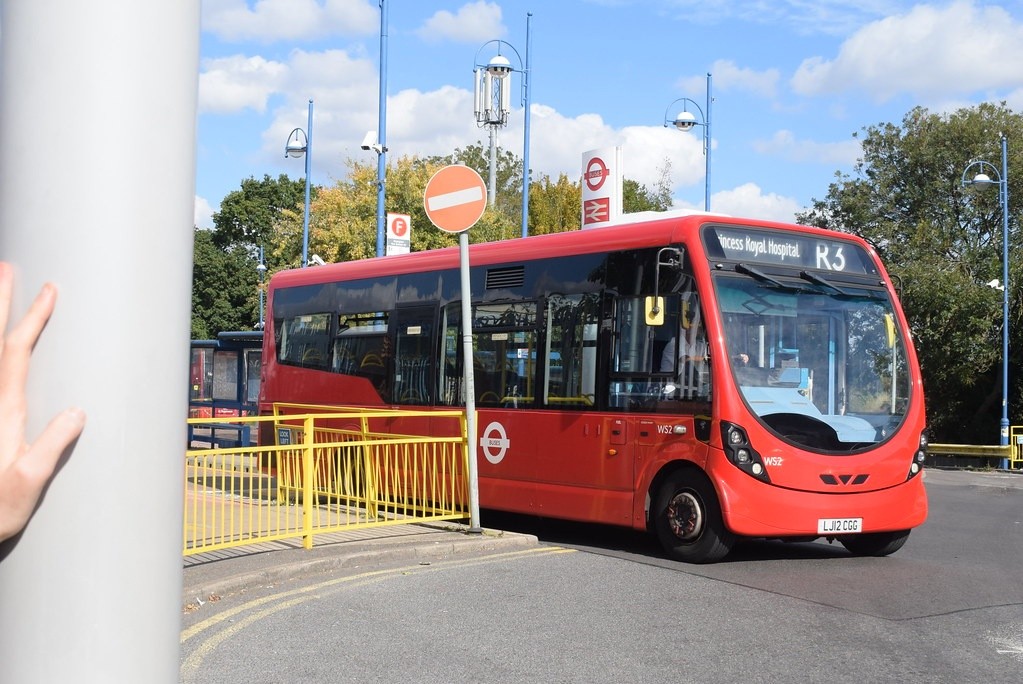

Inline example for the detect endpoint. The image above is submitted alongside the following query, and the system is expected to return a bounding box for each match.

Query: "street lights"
[663,69,714,212]
[471,11,533,237]
[281,98,314,267]
[960,134,1010,472]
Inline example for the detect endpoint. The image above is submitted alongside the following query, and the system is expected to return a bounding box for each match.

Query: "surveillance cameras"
[986,279,999,288]
[361,130,377,150]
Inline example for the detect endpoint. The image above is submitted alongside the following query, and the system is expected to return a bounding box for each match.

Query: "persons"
[656,310,749,393]
[1,259,84,547]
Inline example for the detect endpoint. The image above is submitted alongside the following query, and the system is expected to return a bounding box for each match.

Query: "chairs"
[303,347,595,406]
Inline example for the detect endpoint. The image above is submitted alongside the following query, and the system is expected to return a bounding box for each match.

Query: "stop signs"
[421,165,489,233]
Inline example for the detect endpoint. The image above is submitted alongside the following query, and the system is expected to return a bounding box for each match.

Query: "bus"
[258,212,929,563]
